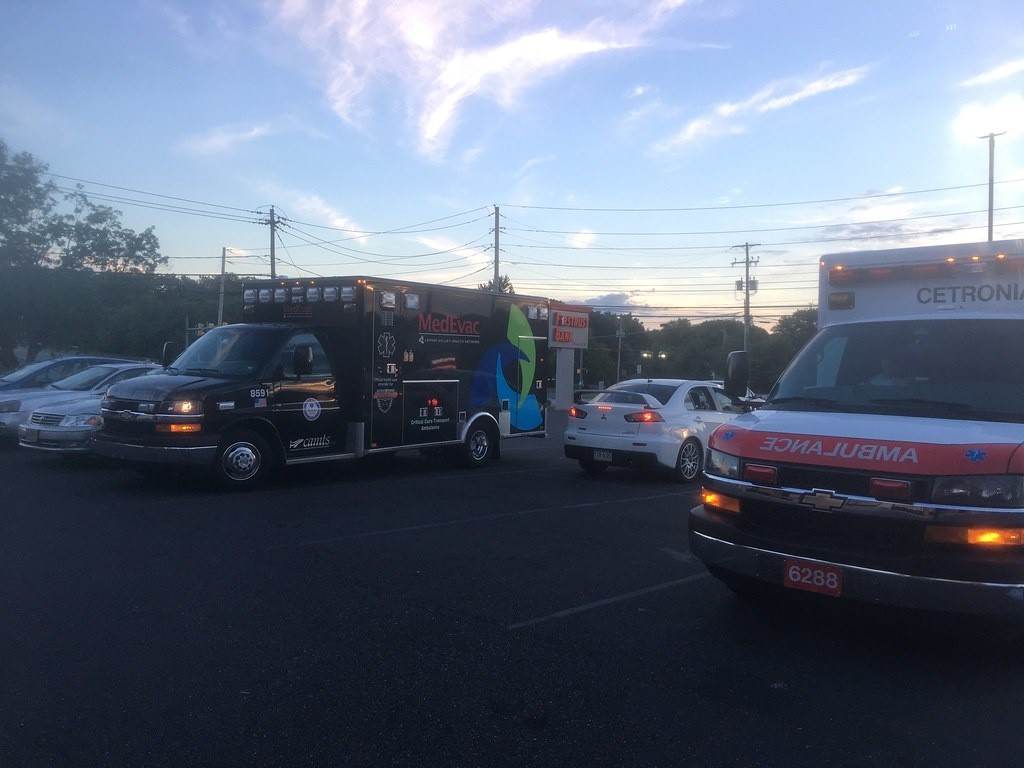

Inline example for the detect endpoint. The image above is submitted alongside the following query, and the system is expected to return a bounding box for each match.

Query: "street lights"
[982,131,1009,240]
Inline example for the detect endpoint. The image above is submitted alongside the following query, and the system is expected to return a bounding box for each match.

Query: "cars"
[563,379,757,482]
[709,381,764,411]
[16,371,165,452]
[0,355,145,391]
[0,364,164,439]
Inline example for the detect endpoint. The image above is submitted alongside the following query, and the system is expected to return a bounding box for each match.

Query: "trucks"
[103,274,550,494]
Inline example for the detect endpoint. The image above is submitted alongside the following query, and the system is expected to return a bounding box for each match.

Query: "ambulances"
[688,238,1024,614]
